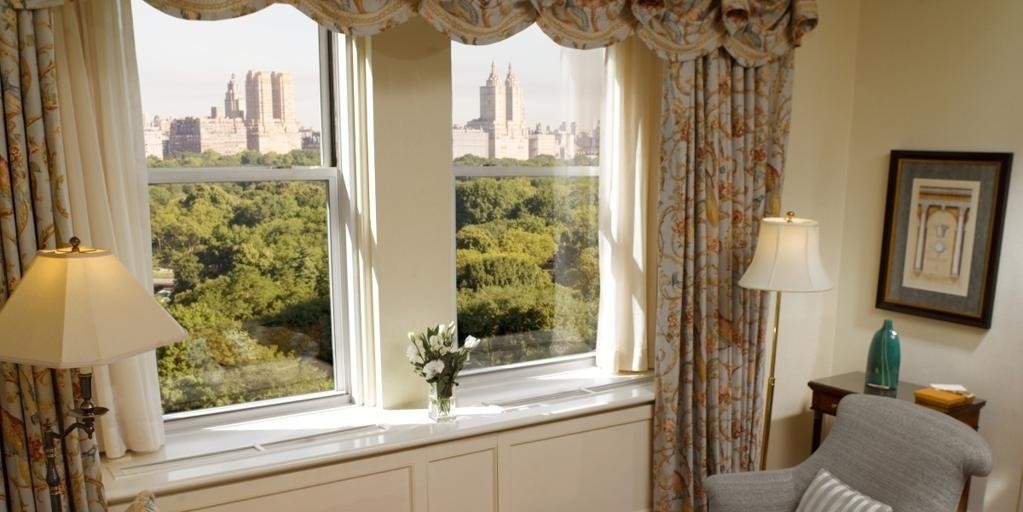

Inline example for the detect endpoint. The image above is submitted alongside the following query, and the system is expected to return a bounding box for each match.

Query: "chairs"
[702,392,994,512]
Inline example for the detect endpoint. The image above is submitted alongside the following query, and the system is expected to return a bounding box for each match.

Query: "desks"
[807,369,986,511]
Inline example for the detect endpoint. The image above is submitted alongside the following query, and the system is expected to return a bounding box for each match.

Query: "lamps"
[0,235,190,511]
[738,211,833,472]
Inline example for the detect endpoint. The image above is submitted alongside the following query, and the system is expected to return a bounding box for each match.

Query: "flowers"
[406,321,481,416]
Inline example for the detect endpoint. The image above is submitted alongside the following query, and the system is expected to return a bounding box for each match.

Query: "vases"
[864,319,901,392]
[426,390,457,422]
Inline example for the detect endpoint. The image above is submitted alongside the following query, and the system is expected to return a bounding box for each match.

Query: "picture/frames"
[873,149,1014,329]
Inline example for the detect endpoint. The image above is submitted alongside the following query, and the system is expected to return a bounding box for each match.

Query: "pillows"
[793,467,893,512]
